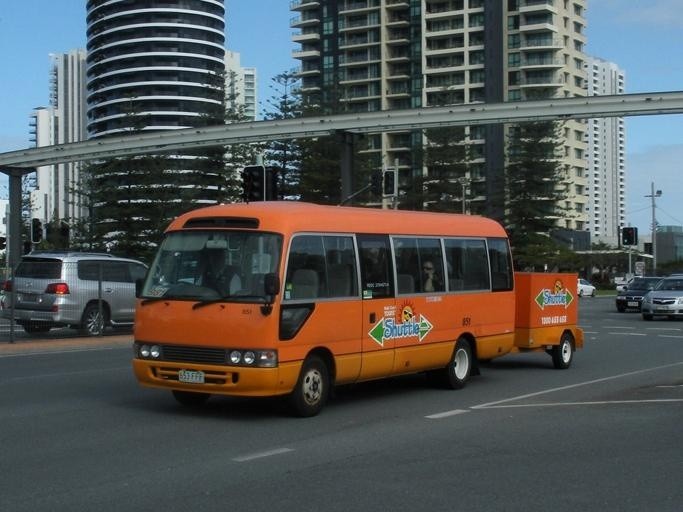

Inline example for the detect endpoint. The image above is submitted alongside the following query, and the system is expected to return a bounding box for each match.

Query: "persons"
[433,247,453,277]
[195,250,242,297]
[415,260,441,292]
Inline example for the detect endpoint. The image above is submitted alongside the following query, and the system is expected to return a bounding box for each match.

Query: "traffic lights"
[264,167,279,200]
[240,165,266,202]
[30,218,42,244]
[0,236,6,250]
[622,227,637,246]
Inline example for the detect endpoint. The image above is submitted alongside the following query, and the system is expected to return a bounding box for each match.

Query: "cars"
[576,278,596,297]
[616,276,664,313]
[640,277,682,320]
[0,249,148,337]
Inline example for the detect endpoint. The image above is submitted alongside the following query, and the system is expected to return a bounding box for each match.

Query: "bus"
[132,198,517,416]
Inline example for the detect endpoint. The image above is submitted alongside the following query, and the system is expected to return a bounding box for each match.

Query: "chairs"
[291,263,464,299]
